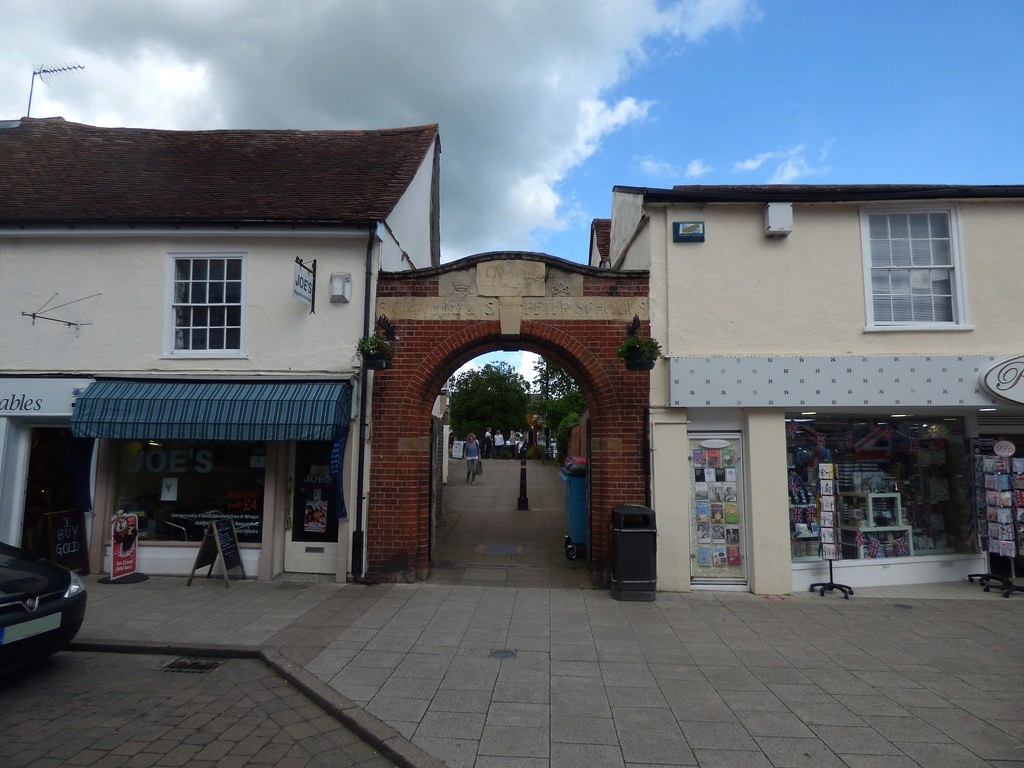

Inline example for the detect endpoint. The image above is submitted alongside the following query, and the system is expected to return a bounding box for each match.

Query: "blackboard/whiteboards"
[213,519,242,568]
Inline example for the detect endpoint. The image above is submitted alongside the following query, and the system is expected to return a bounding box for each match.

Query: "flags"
[817,434,825,449]
[786,421,799,438]
[907,438,919,455]
[806,505,814,521]
[793,507,802,523]
[881,428,891,441]
[788,477,798,493]
[845,433,855,448]
[896,539,907,555]
[867,538,880,558]
[855,529,865,545]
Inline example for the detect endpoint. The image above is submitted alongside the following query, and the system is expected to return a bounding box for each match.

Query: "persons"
[509,430,516,460]
[493,430,505,459]
[698,525,708,538]
[463,434,481,485]
[728,530,738,543]
[485,427,493,458]
[726,487,736,501]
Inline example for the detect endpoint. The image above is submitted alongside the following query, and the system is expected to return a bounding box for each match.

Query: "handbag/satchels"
[475,461,482,476]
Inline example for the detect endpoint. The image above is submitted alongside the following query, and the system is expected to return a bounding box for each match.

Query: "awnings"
[70,382,352,441]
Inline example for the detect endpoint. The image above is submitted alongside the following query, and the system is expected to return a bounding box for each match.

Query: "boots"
[471,473,475,485]
[466,472,471,483]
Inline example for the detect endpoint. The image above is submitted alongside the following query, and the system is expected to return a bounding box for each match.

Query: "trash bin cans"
[558,455,587,561]
[610,504,658,602]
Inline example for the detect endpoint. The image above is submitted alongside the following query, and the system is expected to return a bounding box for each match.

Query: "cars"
[0,541,87,698]
